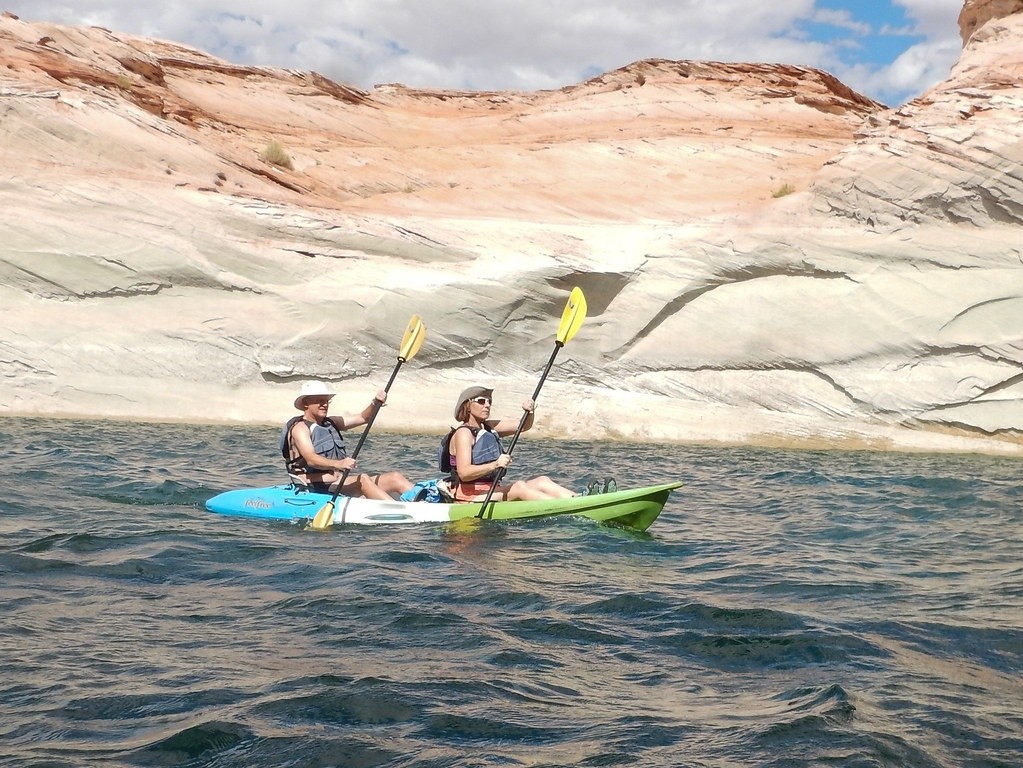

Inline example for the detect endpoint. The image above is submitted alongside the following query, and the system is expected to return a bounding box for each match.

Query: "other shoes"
[571,480,599,497]
[602,477,620,493]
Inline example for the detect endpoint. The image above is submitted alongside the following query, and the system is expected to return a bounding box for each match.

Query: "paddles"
[312,314,428,529]
[473,286,589,519]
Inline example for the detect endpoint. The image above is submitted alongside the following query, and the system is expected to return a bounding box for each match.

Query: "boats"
[201,473,686,535]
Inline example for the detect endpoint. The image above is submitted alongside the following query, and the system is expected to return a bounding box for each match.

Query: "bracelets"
[371,398,387,407]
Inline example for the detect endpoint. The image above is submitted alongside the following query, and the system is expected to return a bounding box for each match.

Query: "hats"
[454,385,494,422]
[293,380,337,411]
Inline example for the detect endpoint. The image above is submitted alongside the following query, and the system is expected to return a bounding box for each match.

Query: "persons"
[287,380,428,502]
[449,386,618,502]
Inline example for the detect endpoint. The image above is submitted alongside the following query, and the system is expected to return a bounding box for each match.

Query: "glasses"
[471,399,492,405]
[305,397,333,404]
